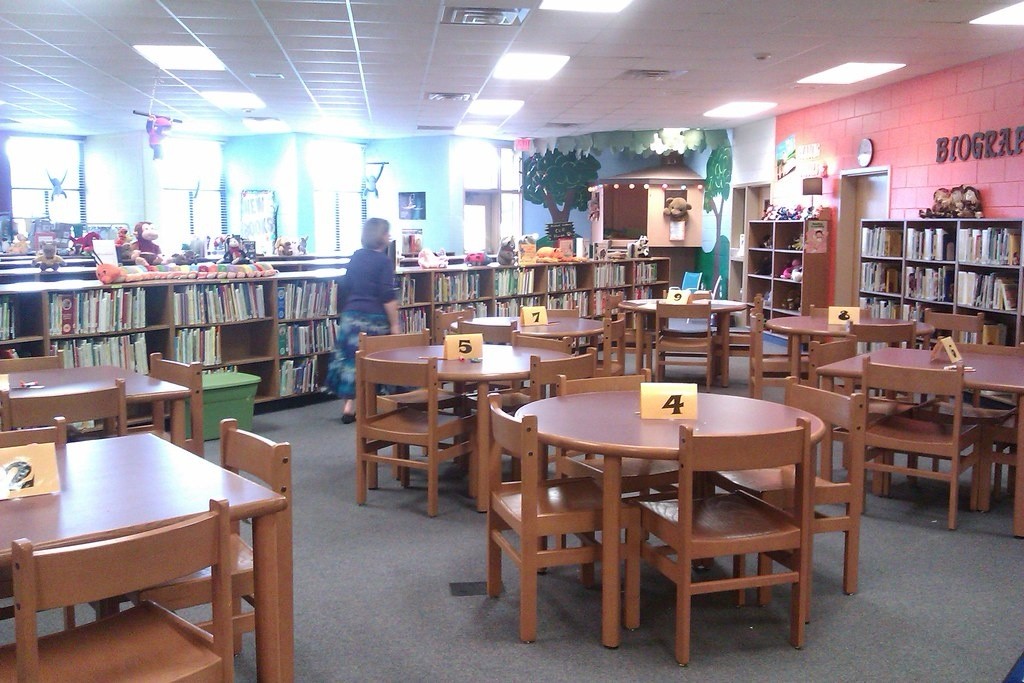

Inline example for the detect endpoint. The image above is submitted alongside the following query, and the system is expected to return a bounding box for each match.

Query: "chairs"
[0,349,294,683]
[355,291,1024,665]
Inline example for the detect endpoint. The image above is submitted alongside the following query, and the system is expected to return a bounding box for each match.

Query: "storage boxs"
[179,372,263,441]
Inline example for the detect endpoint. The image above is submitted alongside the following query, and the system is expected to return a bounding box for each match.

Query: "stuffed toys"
[665,197,693,222]
[419,235,589,267]
[363,163,385,199]
[47,171,69,202]
[6,222,309,284]
[756,204,828,311]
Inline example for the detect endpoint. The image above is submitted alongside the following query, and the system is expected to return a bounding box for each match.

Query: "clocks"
[856,138,873,166]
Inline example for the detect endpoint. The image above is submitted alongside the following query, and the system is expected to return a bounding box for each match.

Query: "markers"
[21,381,38,387]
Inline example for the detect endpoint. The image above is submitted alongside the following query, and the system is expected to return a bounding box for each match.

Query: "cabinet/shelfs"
[0,255,673,450]
[745,220,831,348]
[860,220,1024,352]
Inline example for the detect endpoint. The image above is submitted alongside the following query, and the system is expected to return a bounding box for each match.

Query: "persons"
[145,117,171,160]
[919,184,983,219]
[324,217,400,424]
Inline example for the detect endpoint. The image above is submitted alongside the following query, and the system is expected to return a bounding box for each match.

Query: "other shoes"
[342,413,357,424]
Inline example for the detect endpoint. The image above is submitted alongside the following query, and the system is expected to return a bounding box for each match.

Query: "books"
[434,263,659,345]
[0,295,19,360]
[49,276,427,430]
[859,226,1021,347]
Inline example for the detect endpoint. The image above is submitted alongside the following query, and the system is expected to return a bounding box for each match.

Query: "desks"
[1,364,193,447]
[0,434,287,683]
[515,391,827,648]
[814,346,1024,538]
[619,299,746,386]
[365,342,570,513]
[451,316,606,378]
[766,318,935,403]
